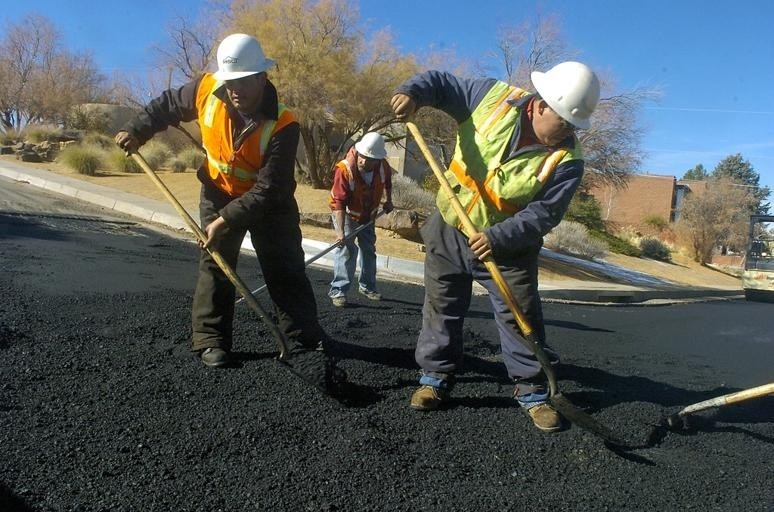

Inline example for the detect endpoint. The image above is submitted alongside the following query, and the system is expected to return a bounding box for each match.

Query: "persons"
[328,131,396,307]
[390,59,601,433]
[114,31,324,368]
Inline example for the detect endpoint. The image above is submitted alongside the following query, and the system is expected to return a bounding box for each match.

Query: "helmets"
[529,62,597,131]
[354,130,388,161]
[213,33,274,82]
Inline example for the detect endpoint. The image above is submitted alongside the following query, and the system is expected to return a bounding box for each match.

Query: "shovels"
[403,114,613,442]
[127,147,351,402]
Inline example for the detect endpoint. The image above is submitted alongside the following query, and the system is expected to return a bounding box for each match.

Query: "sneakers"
[358,287,382,301]
[410,384,450,412]
[200,348,230,368]
[525,403,563,432]
[332,297,347,307]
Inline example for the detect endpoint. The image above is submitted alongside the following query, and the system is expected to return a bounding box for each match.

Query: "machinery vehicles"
[741,215,774,302]
